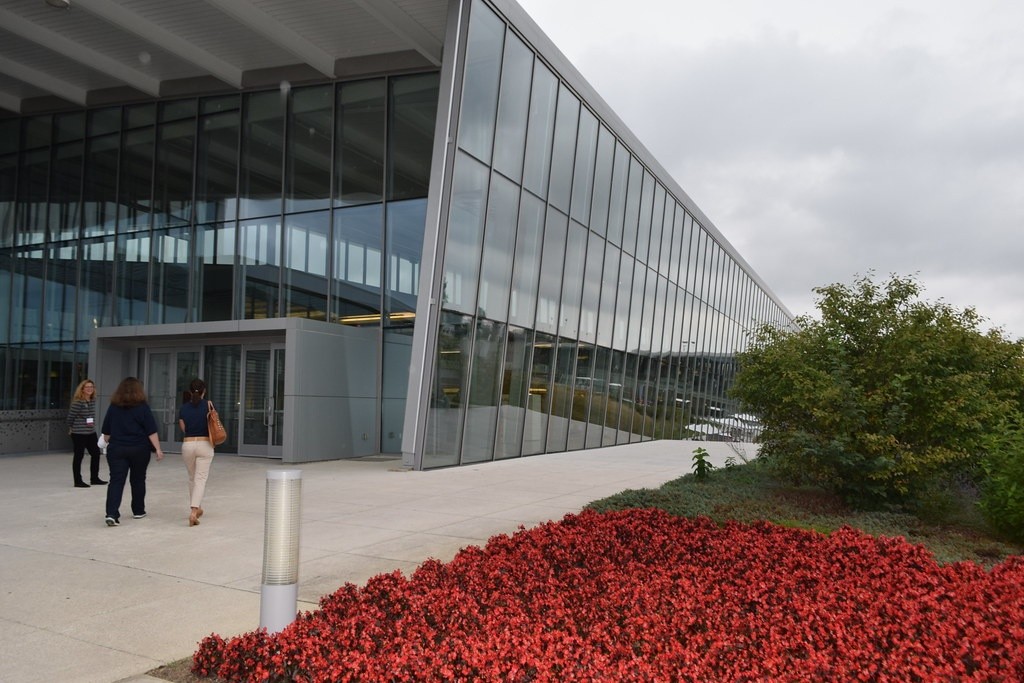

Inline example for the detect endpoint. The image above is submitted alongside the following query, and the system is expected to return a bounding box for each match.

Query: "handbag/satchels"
[207,401,227,448]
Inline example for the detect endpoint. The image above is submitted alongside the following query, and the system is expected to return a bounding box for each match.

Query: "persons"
[64,380,108,488]
[179,379,215,526]
[102,377,163,525]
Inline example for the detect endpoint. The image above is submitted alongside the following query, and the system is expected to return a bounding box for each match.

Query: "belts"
[183,436,209,441]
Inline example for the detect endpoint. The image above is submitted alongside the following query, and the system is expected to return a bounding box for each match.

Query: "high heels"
[197,505,203,518]
[189,515,199,526]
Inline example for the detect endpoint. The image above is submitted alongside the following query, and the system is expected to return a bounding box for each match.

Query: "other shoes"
[91,478,108,485]
[133,511,146,519]
[105,515,120,526]
[75,481,90,487]
